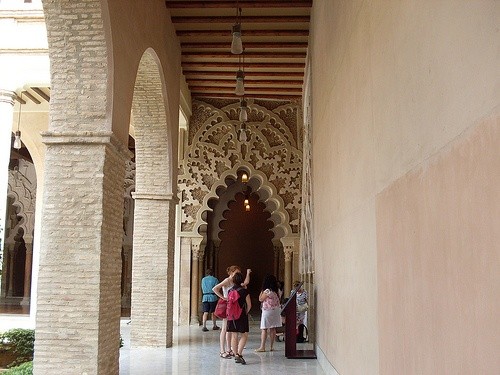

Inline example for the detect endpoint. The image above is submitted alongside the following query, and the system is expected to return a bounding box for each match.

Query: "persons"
[276,280,284,300]
[240,268,252,289]
[289,280,310,342]
[253,275,282,353]
[226,271,251,364]
[201,268,221,331]
[274,297,291,342]
[211,265,242,358]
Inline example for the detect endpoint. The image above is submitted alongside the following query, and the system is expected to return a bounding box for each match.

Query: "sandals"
[226,350,235,356]
[219,351,231,359]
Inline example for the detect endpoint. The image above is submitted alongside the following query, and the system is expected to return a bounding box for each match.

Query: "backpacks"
[227,287,245,320]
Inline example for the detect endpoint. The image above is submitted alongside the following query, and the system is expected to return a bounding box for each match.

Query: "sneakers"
[234,353,246,365]
[255,348,265,352]
[270,348,273,351]
[213,326,220,330]
[201,327,210,332]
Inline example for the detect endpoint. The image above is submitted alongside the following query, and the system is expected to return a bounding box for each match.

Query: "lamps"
[244,195,249,206]
[239,122,247,142]
[239,96,247,120]
[231,0,243,54]
[242,171,248,184]
[246,205,250,211]
[236,54,244,95]
[14,92,22,149]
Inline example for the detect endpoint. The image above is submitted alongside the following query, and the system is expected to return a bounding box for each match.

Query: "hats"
[293,281,300,286]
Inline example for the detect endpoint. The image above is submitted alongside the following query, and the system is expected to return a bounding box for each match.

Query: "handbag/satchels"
[215,298,228,318]
